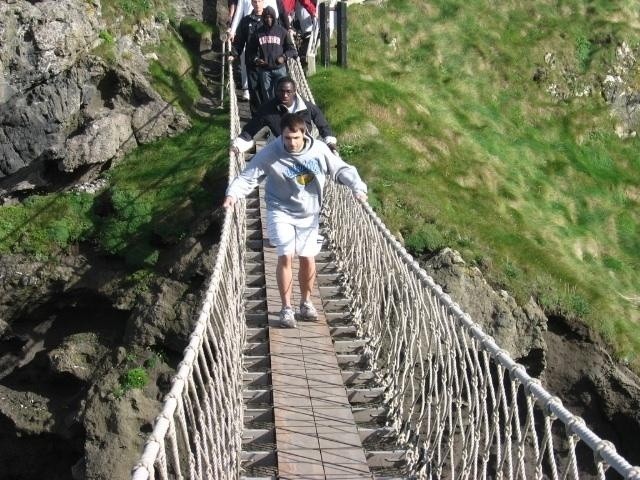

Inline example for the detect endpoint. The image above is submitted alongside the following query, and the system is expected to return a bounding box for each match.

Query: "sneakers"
[300,301,319,321]
[279,306,297,329]
[242,89,250,102]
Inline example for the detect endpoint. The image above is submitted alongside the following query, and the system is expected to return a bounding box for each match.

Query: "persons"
[222,0,320,140]
[219,113,369,329]
[228,76,339,249]
